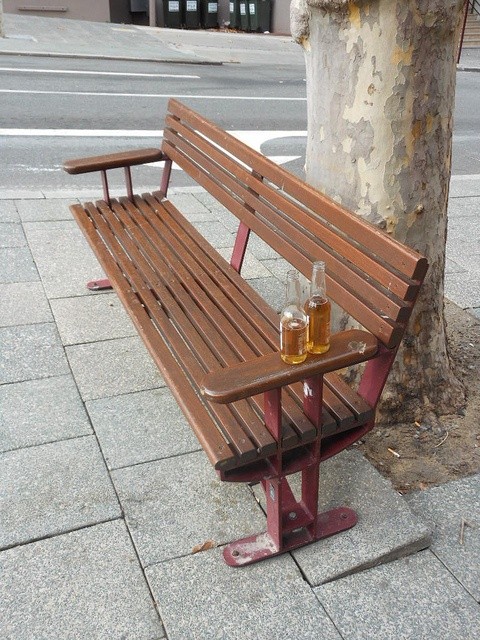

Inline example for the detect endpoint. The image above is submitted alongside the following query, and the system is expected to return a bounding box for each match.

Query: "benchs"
[63,99,429,567]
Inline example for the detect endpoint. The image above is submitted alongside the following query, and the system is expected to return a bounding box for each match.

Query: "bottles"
[304,260,331,354]
[279,270,310,365]
